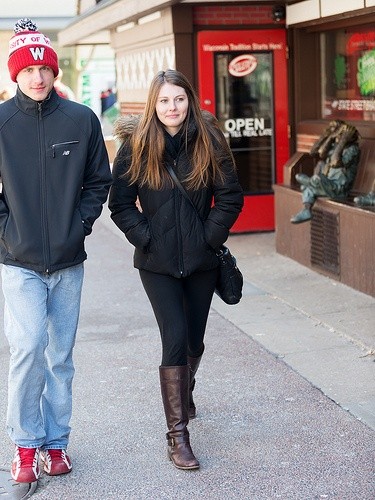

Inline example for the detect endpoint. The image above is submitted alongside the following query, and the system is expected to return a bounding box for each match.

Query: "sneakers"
[11,444,40,483]
[39,449,72,476]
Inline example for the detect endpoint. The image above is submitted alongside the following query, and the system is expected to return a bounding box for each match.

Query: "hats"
[7,18,59,83]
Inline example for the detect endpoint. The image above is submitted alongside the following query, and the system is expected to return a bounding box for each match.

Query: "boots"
[159,364,200,469]
[186,343,205,420]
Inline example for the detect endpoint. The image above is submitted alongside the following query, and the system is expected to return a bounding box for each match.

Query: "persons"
[353,177,375,206]
[108,70,245,470]
[0,18,113,500]
[101,89,116,115]
[290,120,361,224]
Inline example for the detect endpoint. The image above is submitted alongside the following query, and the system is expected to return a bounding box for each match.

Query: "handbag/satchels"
[214,244,243,305]
